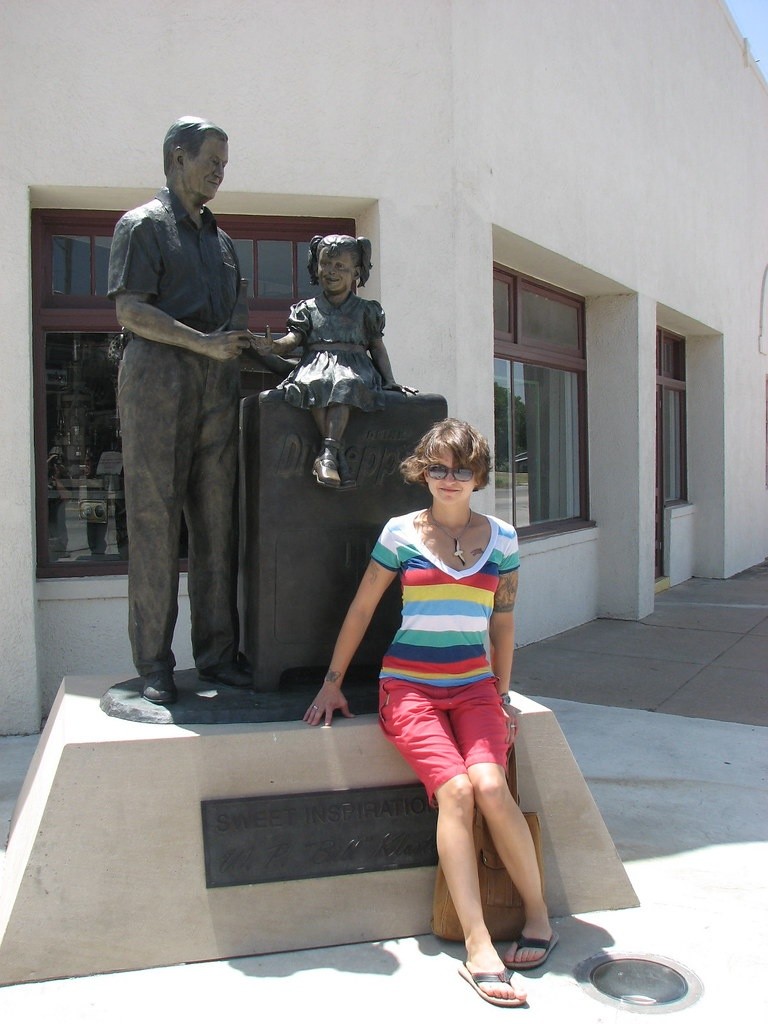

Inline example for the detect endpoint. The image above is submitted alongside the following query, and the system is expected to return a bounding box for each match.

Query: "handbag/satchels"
[431,742,545,941]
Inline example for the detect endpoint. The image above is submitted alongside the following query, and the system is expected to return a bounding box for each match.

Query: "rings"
[313,706,318,709]
[509,725,515,727]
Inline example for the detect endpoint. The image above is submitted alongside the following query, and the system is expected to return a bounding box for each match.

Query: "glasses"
[426,462,479,482]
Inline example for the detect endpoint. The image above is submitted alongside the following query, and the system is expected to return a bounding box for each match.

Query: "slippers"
[501,927,560,969]
[458,961,528,1007]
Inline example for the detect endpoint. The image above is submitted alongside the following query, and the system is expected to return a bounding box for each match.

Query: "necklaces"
[430,506,472,566]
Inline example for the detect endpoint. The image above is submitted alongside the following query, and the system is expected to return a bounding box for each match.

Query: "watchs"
[500,694,510,704]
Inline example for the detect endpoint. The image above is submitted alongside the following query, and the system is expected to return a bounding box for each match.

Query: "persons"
[106,114,298,703]
[302,416,559,1007]
[249,234,419,492]
